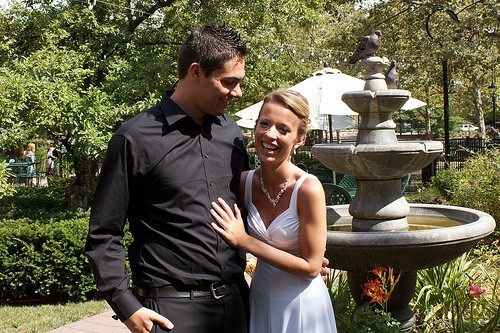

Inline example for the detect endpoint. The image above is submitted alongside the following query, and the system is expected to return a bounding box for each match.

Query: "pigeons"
[384,60,400,89]
[349,30,383,64]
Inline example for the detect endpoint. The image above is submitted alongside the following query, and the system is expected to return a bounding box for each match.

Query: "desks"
[9,161,33,187]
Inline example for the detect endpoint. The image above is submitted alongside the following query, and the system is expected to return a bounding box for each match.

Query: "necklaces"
[259,164,295,208]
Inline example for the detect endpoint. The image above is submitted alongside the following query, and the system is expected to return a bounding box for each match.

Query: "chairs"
[323,184,351,204]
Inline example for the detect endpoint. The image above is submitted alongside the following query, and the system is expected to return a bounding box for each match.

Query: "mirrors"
[26,162,42,187]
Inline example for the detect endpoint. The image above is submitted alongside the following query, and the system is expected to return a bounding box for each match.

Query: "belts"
[132,273,245,299]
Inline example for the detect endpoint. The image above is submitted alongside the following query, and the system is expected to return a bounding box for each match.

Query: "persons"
[23,143,37,187]
[210,90,339,333]
[83,19,331,333]
[46,140,59,186]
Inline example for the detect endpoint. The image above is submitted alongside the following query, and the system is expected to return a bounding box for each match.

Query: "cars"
[457,124,479,132]
